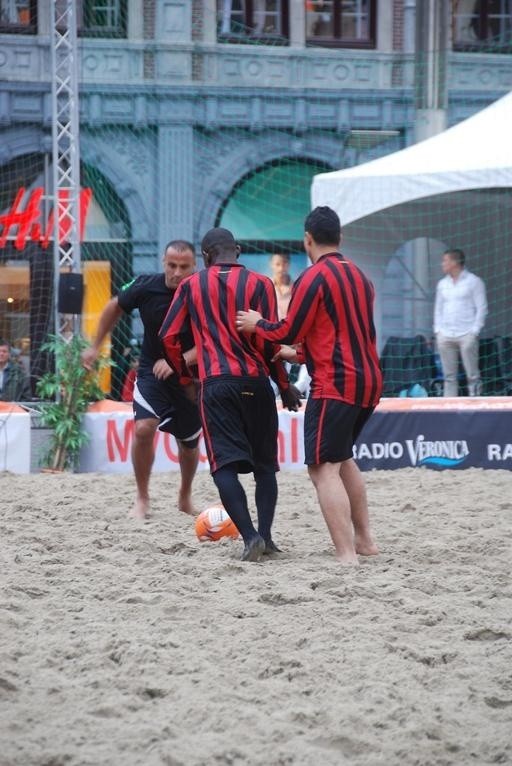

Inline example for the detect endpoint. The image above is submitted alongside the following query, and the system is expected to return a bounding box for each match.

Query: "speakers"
[58,272,84,314]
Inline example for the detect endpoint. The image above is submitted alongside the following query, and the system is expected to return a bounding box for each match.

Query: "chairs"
[375,332,511,396]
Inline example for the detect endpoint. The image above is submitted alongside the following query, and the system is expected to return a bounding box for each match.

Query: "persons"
[264,251,299,379]
[1,340,32,403]
[82,241,202,521]
[434,248,488,398]
[235,206,383,565]
[159,228,304,562]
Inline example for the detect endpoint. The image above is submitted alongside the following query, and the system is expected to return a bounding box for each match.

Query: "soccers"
[195,505,239,544]
